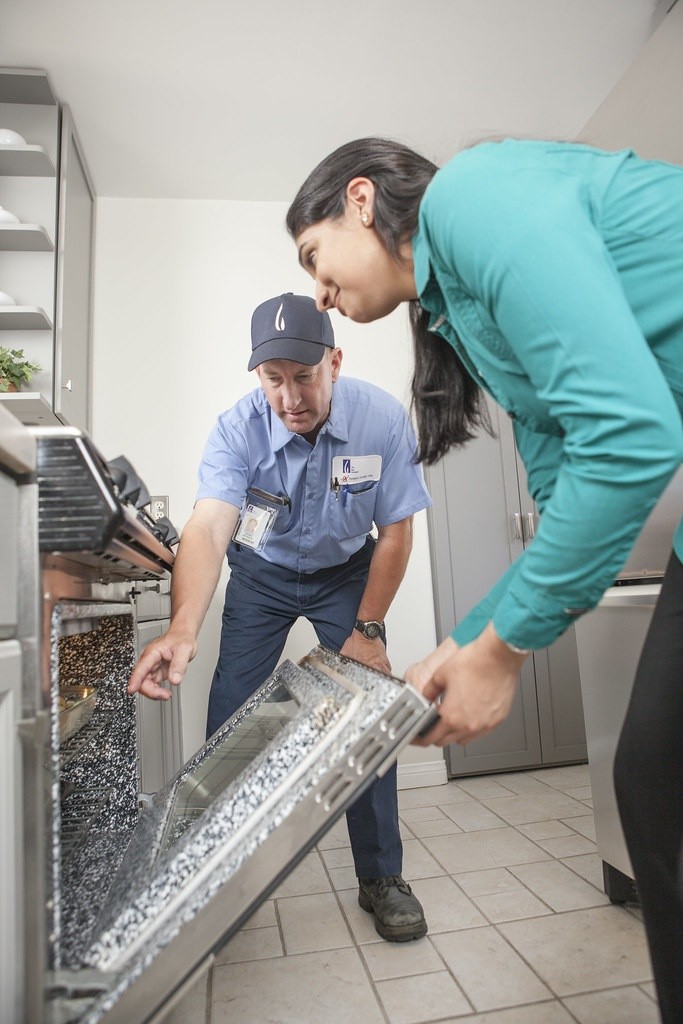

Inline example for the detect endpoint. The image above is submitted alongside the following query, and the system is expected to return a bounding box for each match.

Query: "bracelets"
[506,642,531,656]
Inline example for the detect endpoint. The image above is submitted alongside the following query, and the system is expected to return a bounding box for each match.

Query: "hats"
[247,292,335,372]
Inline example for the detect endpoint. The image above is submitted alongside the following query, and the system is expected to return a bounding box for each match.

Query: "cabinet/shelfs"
[0,66,99,431]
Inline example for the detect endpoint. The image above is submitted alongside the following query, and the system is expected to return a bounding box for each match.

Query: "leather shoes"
[358,873,428,942]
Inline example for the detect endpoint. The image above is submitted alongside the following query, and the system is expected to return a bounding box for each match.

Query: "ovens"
[9,421,445,1024]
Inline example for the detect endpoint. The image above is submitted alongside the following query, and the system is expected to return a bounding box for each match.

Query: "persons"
[285,137,683,1024]
[245,519,257,532]
[130,292,433,943]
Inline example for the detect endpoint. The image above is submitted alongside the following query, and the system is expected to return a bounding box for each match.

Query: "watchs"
[354,619,384,639]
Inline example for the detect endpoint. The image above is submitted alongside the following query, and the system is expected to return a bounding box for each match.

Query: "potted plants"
[0,347,41,392]
